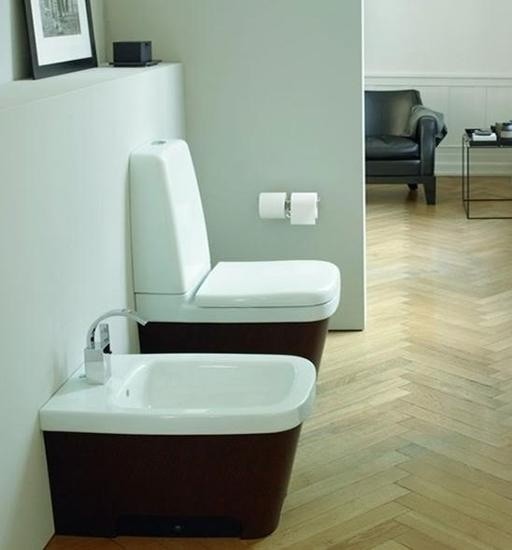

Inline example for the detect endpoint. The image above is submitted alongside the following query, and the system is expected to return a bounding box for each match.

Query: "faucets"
[84,308,149,385]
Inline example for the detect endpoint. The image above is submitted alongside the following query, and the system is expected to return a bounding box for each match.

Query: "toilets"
[128,137,343,373]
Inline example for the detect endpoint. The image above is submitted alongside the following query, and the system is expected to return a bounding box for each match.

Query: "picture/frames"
[24,0,98,80]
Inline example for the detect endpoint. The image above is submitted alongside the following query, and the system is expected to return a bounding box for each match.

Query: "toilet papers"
[290,192,319,226]
[258,192,288,220]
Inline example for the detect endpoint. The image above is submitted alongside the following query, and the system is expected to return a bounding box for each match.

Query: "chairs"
[365,88,438,205]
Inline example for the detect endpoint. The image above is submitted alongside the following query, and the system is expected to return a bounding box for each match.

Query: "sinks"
[40,353,318,438]
